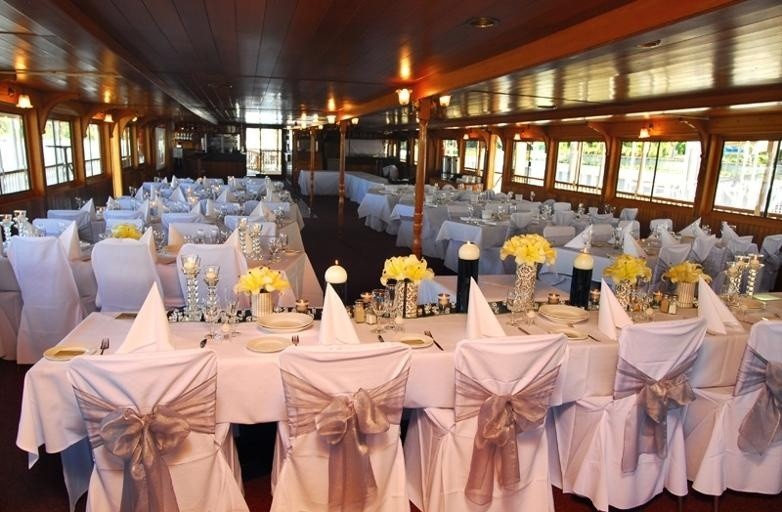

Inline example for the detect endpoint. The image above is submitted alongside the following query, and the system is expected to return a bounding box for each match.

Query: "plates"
[721,295,766,312]
[537,303,591,340]
[243,299,315,354]
[42,345,87,362]
[394,333,433,348]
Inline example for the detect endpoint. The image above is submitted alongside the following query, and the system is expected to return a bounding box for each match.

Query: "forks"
[99,338,110,354]
[423,327,444,354]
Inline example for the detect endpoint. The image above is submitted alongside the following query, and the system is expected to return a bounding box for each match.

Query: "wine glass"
[200,286,241,341]
[371,287,392,334]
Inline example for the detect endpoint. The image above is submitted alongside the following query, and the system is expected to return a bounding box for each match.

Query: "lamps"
[463,129,472,140]
[439,95,452,108]
[100,111,114,123]
[394,88,420,115]
[8,87,34,109]
[639,123,654,139]
[351,117,360,129]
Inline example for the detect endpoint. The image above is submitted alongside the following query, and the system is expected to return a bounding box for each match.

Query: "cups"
[0,174,295,260]
[386,172,711,255]
[510,310,534,327]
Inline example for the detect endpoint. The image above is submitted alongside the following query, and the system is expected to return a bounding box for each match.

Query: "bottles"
[353,300,366,323]
[652,291,678,315]
